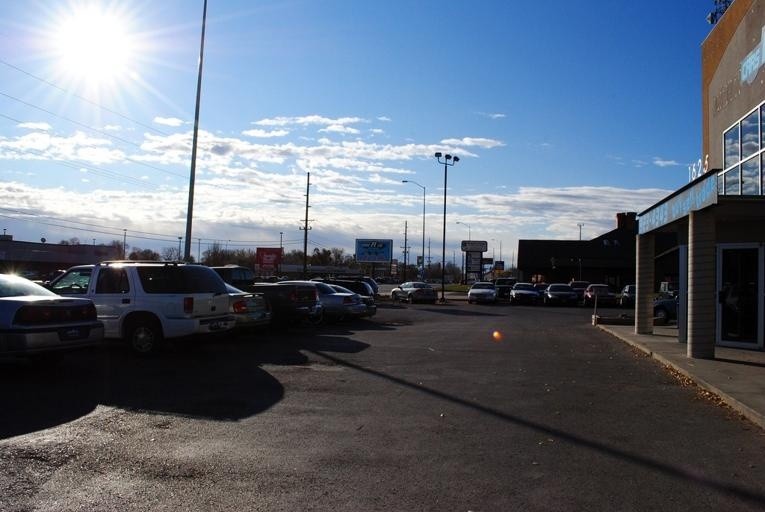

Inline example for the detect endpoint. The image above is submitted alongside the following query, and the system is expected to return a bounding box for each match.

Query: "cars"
[224,283,273,329]
[390,282,438,304]
[0,271,96,371]
[209,264,379,332]
[468,277,679,325]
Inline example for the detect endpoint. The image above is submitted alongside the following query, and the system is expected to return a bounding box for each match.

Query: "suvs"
[41,261,237,357]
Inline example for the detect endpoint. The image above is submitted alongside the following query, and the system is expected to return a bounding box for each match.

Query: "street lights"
[403,181,425,282]
[435,153,459,303]
[456,222,471,241]
